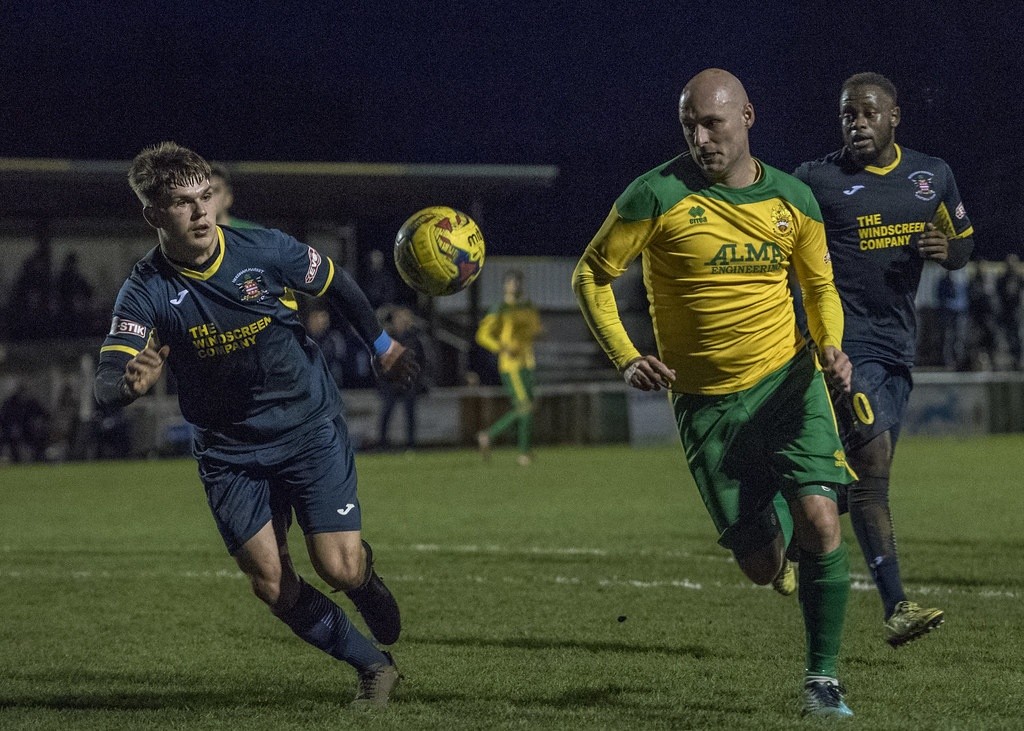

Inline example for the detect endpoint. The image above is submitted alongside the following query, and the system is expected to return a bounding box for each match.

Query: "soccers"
[395,205,489,298]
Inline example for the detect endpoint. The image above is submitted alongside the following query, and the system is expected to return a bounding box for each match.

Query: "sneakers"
[352,571,401,645]
[883,601,945,650]
[350,650,405,704]
[772,557,796,596]
[802,676,853,717]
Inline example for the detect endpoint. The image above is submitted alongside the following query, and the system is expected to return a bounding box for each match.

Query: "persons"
[2,228,500,463]
[476,271,542,465]
[933,254,1024,373]
[92,145,426,710]
[571,67,860,717]
[792,74,978,647]
[374,306,433,454]
